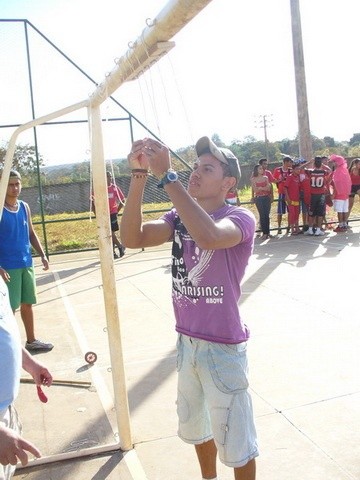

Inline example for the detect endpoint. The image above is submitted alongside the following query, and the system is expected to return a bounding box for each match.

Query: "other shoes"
[113,251,118,258]
[334,226,346,232]
[278,227,282,234]
[295,229,303,233]
[118,245,125,256]
[25,339,54,351]
[291,232,298,235]
[315,230,325,235]
[287,227,291,233]
[304,229,314,235]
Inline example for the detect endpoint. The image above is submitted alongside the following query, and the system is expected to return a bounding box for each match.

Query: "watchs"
[158,170,178,189]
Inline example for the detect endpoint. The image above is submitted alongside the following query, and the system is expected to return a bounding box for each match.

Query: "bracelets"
[129,166,149,179]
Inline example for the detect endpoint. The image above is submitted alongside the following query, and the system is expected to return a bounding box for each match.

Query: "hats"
[196,136,242,193]
[10,170,21,179]
[299,159,307,163]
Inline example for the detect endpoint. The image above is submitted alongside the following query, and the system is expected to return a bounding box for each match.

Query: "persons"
[251,155,360,238]
[91,171,126,259]
[120,136,260,480]
[0,169,53,352]
[0,272,52,466]
